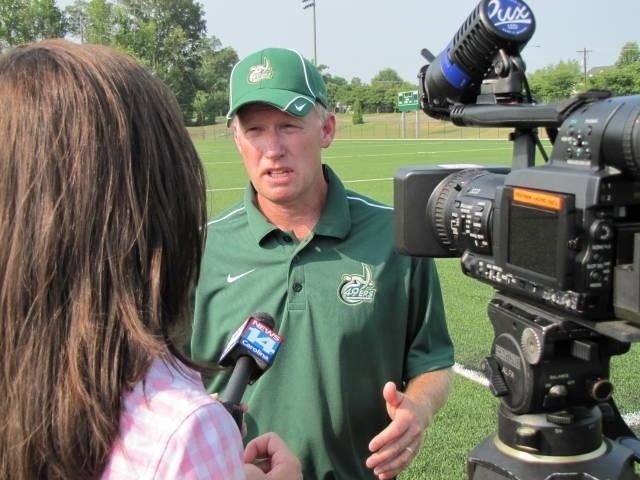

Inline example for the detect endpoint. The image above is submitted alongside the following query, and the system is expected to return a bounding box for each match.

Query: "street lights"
[301,0,318,69]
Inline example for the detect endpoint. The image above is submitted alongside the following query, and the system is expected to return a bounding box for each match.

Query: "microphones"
[216,311,284,431]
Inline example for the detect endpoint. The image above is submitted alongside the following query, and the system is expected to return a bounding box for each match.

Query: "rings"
[406,447,418,459]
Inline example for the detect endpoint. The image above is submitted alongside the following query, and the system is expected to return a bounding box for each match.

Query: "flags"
[300,0,317,12]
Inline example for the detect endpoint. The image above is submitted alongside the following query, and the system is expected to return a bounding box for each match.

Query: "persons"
[191,46,455,480]
[0,39,306,479]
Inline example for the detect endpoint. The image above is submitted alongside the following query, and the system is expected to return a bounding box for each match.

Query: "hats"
[225,47,328,128]
